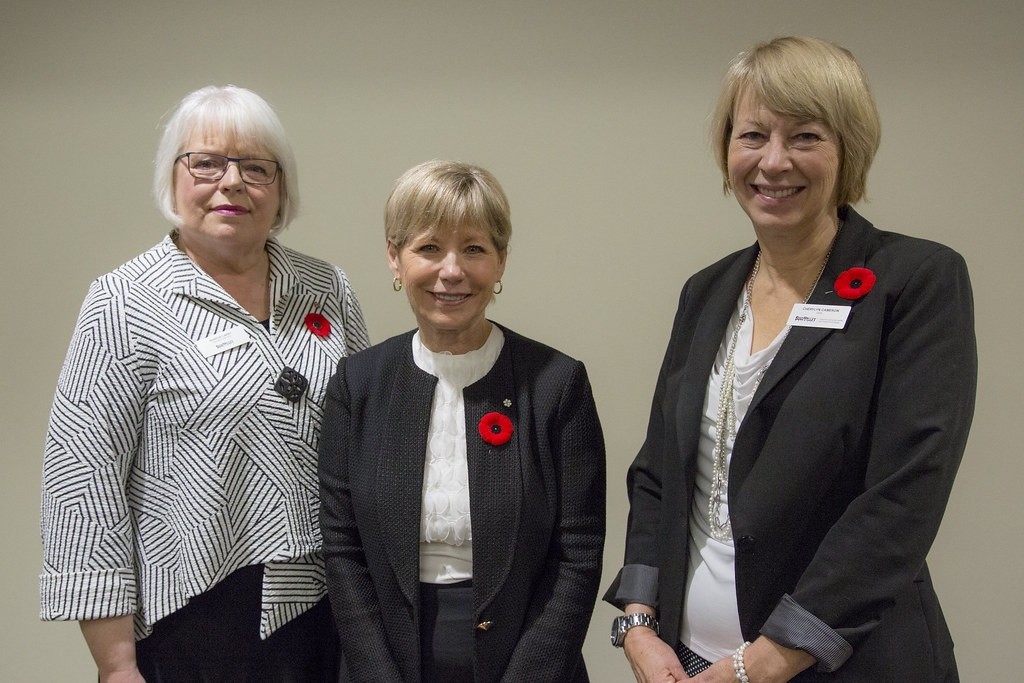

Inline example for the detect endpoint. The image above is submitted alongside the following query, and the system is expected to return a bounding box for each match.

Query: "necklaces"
[706,244,836,542]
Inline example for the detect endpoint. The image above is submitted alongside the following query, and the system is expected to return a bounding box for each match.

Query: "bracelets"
[730,640,754,683]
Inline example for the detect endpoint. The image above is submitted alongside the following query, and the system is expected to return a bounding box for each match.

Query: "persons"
[600,32,980,683]
[35,83,375,683]
[316,156,609,683]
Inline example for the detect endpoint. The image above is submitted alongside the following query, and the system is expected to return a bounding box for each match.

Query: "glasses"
[175,151,284,185]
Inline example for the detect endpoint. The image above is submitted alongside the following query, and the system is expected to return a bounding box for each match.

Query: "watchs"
[609,612,663,654]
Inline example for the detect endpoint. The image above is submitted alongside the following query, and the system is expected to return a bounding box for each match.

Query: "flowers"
[313,301,321,308]
[478,411,513,445]
[503,399,512,407]
[834,266,875,300]
[304,312,331,336]
